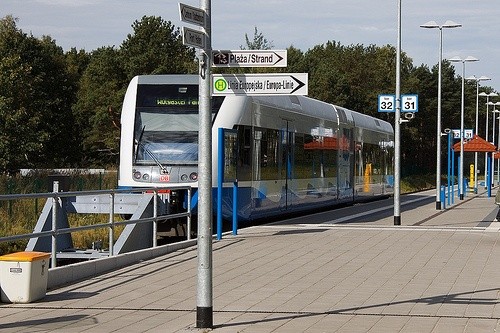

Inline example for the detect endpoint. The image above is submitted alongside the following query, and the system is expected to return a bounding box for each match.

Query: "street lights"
[419,19,462,211]
[485,102,500,188]
[466,75,491,195]
[477,91,498,190]
[447,56,480,201]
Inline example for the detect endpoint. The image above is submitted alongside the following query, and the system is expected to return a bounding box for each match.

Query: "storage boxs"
[0,252,51,303]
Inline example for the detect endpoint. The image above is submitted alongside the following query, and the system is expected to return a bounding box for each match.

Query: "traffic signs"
[177,1,309,97]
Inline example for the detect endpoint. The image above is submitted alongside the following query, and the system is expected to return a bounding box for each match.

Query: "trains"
[117,74,395,236]
